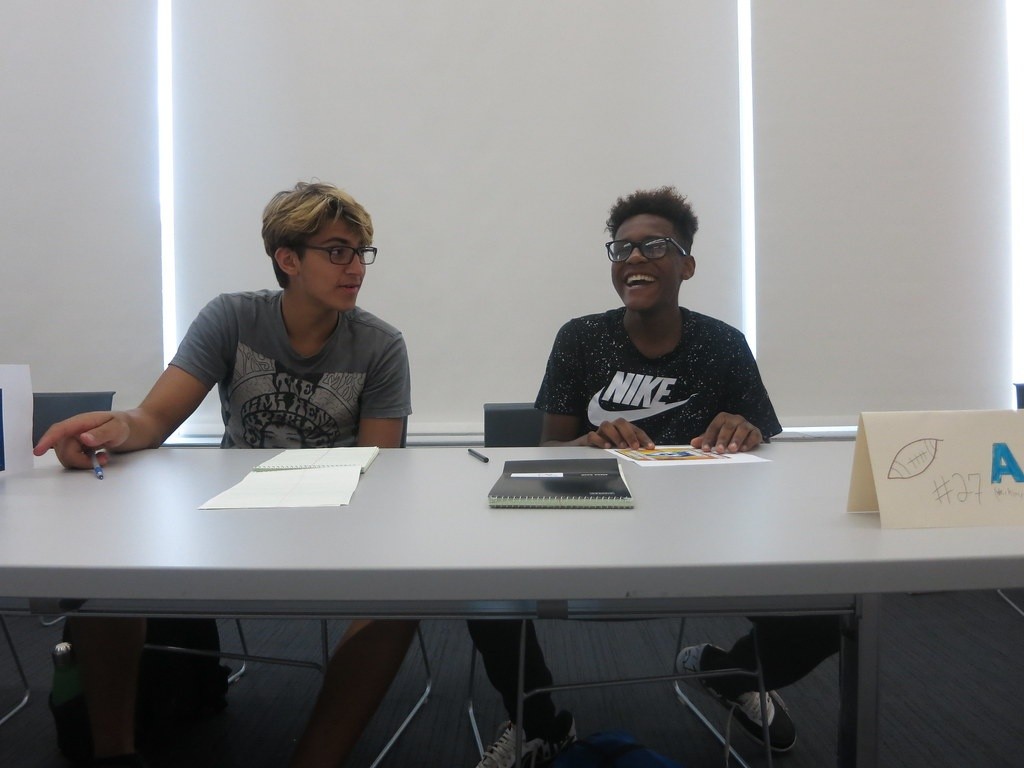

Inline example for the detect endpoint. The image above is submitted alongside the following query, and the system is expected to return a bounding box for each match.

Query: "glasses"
[605,237,687,263]
[296,245,377,265]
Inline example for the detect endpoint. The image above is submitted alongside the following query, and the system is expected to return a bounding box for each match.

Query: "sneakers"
[478,714,576,768]
[676,643,798,766]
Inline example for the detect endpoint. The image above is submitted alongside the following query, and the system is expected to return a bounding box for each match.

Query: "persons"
[33,179,424,768]
[468,184,846,768]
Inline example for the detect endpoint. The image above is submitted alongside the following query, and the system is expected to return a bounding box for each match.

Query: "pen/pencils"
[468,448,489,463]
[87,445,104,478]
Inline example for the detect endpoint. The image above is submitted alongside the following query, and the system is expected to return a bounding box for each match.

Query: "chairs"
[464,400,773,767]
[30,390,437,766]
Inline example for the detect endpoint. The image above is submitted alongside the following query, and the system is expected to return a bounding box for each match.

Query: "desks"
[0,439,1024,768]
